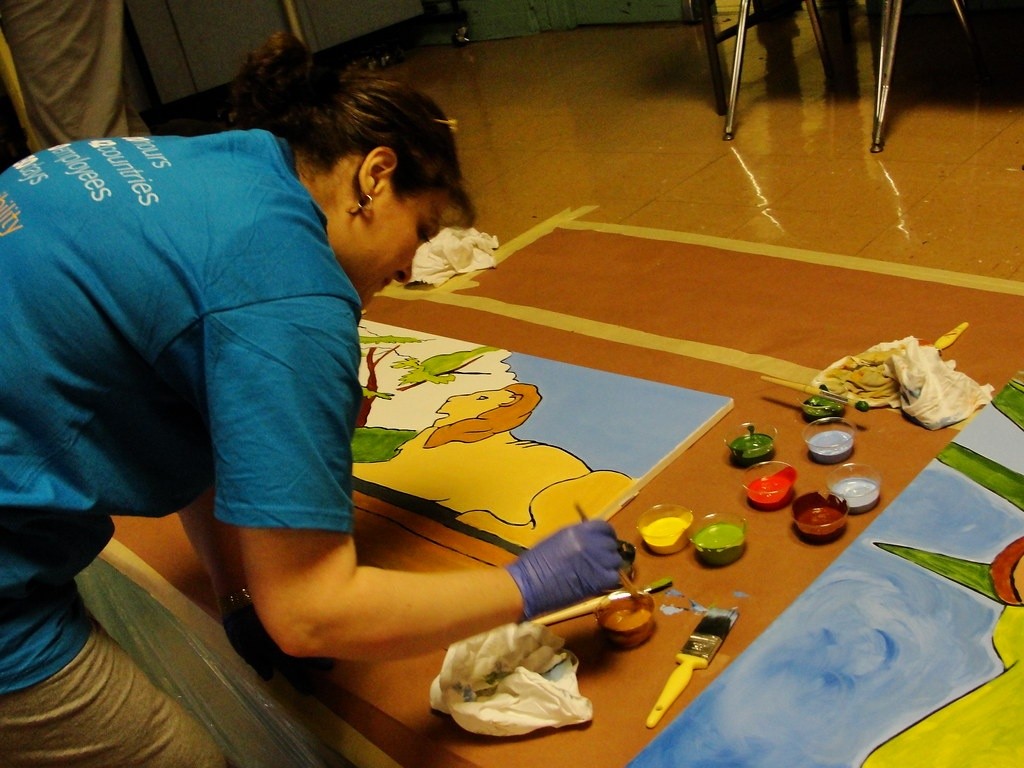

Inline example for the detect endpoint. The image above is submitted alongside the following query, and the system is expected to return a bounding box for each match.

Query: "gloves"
[506,519,626,619]
[220,602,341,697]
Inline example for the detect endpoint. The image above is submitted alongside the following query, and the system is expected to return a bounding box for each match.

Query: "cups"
[725,422,777,463]
[637,504,693,554]
[689,512,747,564]
[826,463,880,513]
[597,589,656,647]
[800,380,849,420]
[741,461,795,510]
[788,490,849,536]
[802,417,857,464]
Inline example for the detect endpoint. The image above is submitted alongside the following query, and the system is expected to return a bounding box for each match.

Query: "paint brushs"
[533,576,672,622]
[760,375,869,412]
[573,502,648,607]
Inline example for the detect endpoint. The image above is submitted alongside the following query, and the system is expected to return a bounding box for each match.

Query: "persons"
[0,31,626,768]
[0,0,150,152]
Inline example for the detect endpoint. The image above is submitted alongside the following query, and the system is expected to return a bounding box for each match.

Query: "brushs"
[644,608,738,729]
